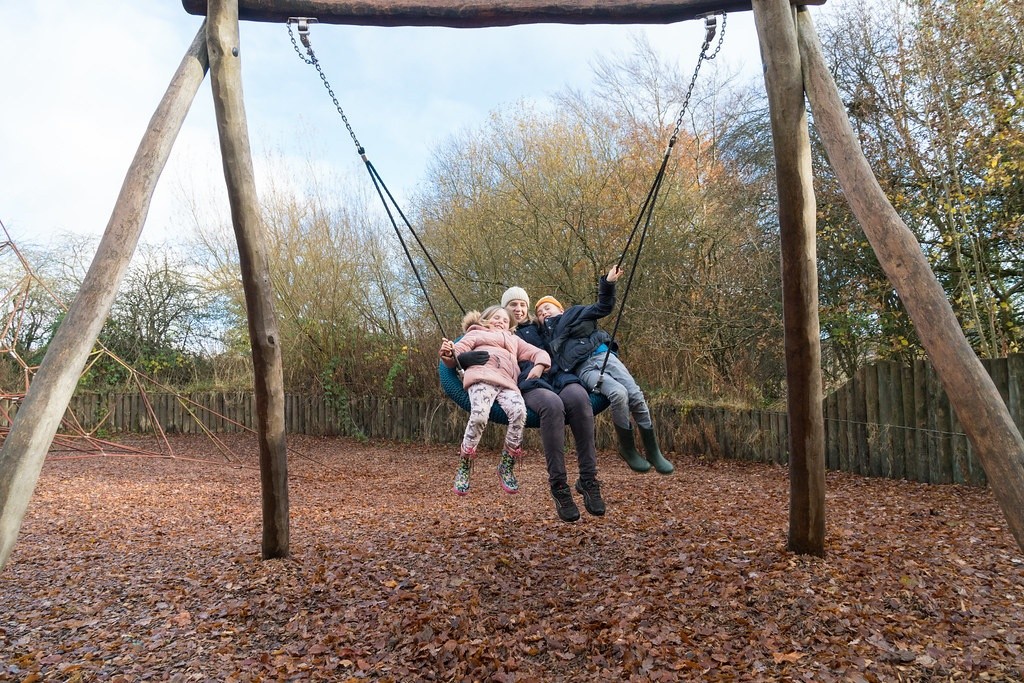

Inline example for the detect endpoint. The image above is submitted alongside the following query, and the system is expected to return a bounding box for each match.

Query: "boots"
[613,420,651,472]
[454,443,478,496]
[497,443,523,493]
[636,421,675,475]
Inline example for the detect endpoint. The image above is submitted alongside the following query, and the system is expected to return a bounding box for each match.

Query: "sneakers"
[575,478,606,515]
[550,485,580,521]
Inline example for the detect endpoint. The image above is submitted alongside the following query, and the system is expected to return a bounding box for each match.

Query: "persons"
[455,286,606,522]
[534,265,674,474]
[439,306,551,496]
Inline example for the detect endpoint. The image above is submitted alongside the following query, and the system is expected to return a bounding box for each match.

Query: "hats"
[501,286,530,309]
[534,295,563,312]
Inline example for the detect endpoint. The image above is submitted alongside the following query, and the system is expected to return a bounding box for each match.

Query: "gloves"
[457,351,490,371]
[569,320,594,339]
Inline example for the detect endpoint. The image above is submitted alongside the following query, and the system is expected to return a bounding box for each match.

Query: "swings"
[288,23,722,426]
